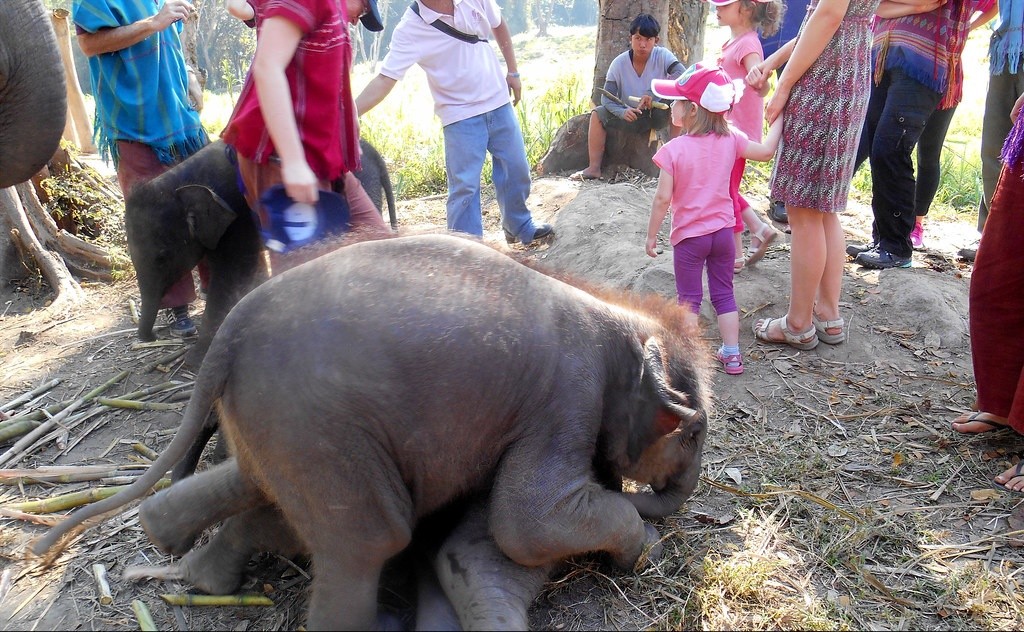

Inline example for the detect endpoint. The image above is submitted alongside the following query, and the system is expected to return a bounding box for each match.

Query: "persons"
[353,0,554,242]
[747,0,883,348]
[644,57,783,374]
[713,0,789,273]
[73,1,212,340]
[846,0,999,271]
[949,86,1023,491]
[220,0,391,282]
[569,14,687,181]
[958,0,1023,262]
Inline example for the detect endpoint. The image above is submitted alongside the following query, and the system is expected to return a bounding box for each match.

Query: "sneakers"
[910,221,924,248]
[958,245,979,260]
[846,242,912,269]
[166,304,198,339]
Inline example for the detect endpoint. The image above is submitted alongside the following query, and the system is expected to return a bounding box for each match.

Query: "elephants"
[124,137,400,366]
[33,227,714,632]
[0,0,68,190]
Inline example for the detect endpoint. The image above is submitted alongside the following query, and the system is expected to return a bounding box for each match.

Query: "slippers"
[949,412,1012,436]
[992,461,1024,498]
[568,169,595,182]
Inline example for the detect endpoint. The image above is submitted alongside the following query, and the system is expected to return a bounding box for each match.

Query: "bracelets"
[507,72,520,77]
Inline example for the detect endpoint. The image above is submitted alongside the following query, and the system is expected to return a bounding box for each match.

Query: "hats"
[650,60,745,115]
[263,185,350,246]
[360,0,384,32]
[707,0,770,7]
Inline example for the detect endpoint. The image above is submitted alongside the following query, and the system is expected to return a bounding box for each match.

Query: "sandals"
[752,316,818,350]
[811,314,845,343]
[716,349,743,375]
[744,222,778,265]
[733,255,743,273]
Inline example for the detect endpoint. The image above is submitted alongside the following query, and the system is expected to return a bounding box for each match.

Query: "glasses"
[358,1,369,18]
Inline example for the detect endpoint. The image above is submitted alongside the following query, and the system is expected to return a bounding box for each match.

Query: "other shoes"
[506,222,551,243]
